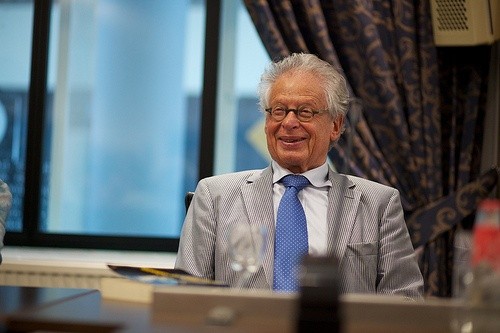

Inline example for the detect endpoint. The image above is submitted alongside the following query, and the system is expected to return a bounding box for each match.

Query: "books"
[100,266,229,305]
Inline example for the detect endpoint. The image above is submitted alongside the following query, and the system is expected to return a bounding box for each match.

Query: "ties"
[272,174,311,293]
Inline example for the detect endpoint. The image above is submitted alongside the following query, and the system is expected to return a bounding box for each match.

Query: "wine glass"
[229,223,263,288]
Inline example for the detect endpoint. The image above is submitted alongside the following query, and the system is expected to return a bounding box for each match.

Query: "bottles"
[460,199,500,333]
[295,255,344,333]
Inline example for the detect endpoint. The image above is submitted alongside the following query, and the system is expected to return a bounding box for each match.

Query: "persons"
[0,180,13,268]
[174,54,428,300]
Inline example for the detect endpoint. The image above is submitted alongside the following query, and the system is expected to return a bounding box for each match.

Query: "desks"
[0,286,147,333]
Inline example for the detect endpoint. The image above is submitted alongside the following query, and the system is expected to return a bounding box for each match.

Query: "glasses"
[264,103,329,122]
